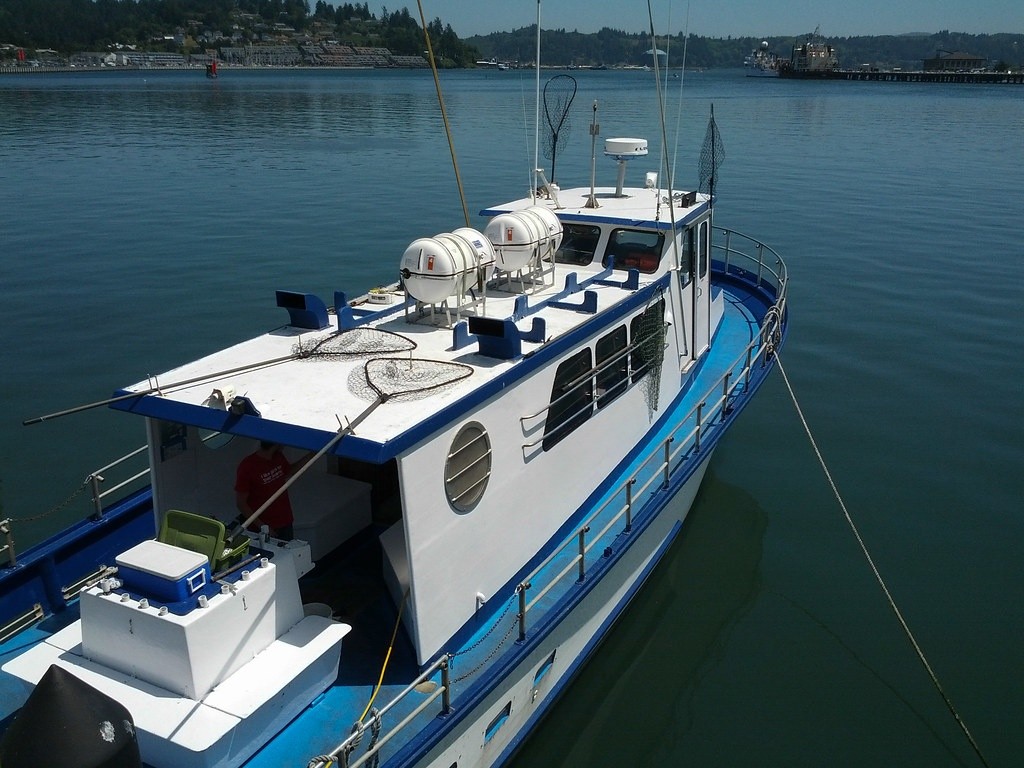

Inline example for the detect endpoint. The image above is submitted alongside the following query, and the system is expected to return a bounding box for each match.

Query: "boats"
[780,25,839,80]
[742,40,779,77]
[0,1,792,768]
[205,62,218,79]
